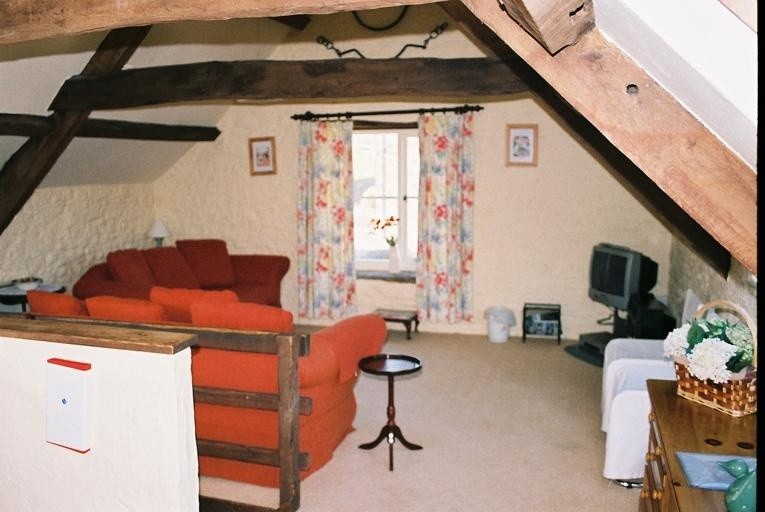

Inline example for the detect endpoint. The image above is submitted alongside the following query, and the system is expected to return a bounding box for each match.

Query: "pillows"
[25,291,86,316]
[140,247,200,289]
[83,297,167,322]
[151,287,239,324]
[176,240,234,288]
[192,301,295,332]
[106,248,155,283]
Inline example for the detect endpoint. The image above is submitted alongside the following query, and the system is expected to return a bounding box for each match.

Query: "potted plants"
[662,300,758,416]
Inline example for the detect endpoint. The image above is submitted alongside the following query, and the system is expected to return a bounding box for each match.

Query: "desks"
[375,309,419,340]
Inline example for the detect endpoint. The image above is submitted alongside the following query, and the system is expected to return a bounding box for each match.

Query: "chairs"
[602,288,704,488]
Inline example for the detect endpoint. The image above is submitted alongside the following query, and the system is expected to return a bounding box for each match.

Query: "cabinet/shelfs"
[637,379,758,512]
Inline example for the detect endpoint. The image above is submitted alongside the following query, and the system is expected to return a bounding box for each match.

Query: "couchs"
[191,315,387,488]
[73,254,290,308]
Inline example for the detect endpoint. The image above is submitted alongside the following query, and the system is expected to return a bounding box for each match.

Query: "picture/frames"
[504,123,538,167]
[248,137,276,177]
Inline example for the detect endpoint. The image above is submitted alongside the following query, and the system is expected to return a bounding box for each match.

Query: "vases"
[388,247,400,274]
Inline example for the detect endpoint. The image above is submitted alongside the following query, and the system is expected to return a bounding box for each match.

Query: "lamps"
[148,221,171,245]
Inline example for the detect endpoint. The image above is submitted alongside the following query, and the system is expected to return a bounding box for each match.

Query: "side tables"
[359,354,422,472]
[0,279,66,315]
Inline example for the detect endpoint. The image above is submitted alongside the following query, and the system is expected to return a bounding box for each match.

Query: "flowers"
[370,216,401,246]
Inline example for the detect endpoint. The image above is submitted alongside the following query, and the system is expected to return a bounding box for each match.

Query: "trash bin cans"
[484,306,518,344]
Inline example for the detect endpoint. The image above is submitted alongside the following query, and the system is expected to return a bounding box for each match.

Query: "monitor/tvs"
[588,243,659,308]
